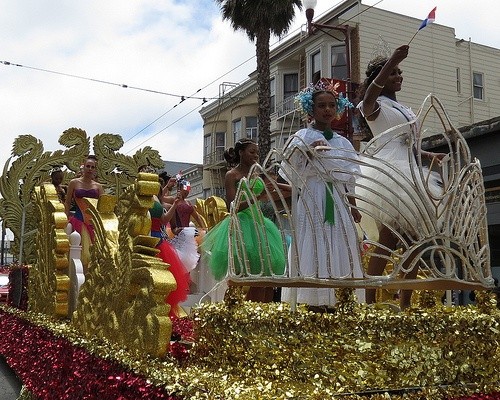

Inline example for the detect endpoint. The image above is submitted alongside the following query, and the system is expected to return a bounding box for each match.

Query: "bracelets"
[372,79,385,89]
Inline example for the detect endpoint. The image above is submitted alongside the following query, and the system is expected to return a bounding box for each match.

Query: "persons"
[278,78,366,313]
[63,155,105,244]
[346,44,447,312]
[200,138,285,281]
[155,171,206,293]
[137,164,191,321]
[50,160,98,217]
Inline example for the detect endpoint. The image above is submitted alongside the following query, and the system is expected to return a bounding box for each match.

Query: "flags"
[182,181,191,191]
[417,6,437,31]
[176,172,182,180]
